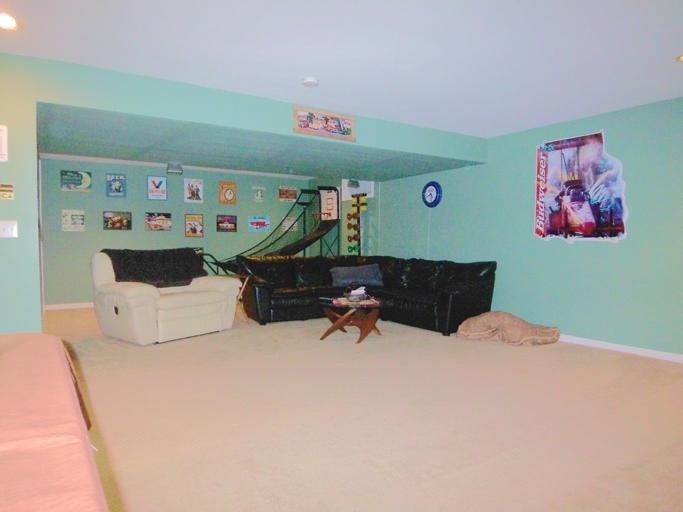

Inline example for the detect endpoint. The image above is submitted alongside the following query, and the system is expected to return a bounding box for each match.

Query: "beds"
[0,332,114,512]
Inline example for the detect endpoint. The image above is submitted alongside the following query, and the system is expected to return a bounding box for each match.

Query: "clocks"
[422,181,442,207]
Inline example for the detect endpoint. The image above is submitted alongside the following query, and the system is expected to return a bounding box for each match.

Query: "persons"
[185,182,202,201]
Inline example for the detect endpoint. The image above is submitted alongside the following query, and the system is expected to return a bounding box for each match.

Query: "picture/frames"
[184,214,237,237]
[146,175,168,201]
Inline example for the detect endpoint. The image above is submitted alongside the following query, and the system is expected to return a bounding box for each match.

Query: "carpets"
[458,310,561,346]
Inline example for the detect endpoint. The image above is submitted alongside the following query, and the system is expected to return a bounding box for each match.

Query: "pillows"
[252,254,461,292]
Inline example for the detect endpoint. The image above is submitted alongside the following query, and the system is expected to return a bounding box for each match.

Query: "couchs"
[231,255,497,337]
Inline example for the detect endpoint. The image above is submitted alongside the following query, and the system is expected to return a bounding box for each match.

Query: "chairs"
[89,248,241,345]
